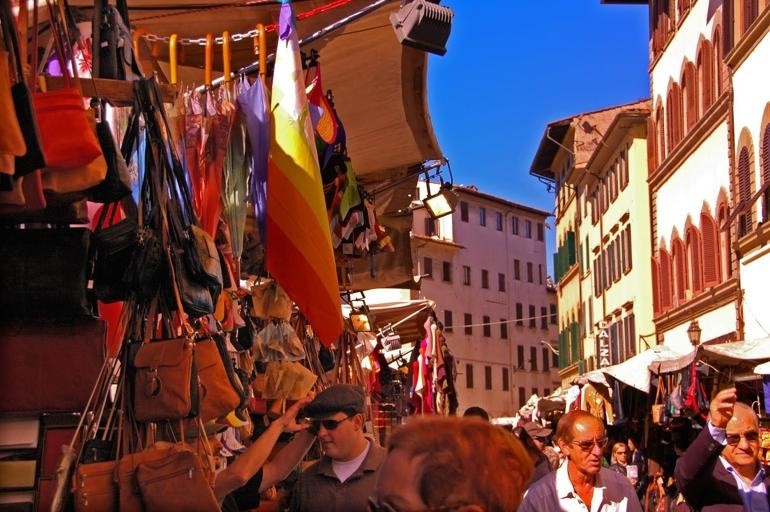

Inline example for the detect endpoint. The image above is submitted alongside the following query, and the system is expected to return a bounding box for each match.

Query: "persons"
[464,386,770,512]
[374,415,533,512]
[169,384,385,511]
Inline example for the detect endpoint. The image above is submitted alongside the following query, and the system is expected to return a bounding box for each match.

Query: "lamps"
[346,289,373,332]
[389,0,453,56]
[688,320,702,345]
[398,358,409,375]
[379,323,402,351]
[422,166,459,220]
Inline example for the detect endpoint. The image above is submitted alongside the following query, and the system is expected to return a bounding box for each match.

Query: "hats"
[184,423,229,439]
[522,422,553,436]
[304,384,365,413]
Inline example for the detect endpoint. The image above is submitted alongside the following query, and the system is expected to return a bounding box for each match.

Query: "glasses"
[572,438,608,451]
[534,437,545,442]
[725,431,759,445]
[310,415,354,433]
[616,451,627,455]
[368,496,458,512]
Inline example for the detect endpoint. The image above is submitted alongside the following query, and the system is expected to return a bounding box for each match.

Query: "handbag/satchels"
[651,383,710,425]
[0,222,114,414]
[129,332,247,420]
[0,49,133,223]
[50,439,221,512]
[93,215,227,317]
